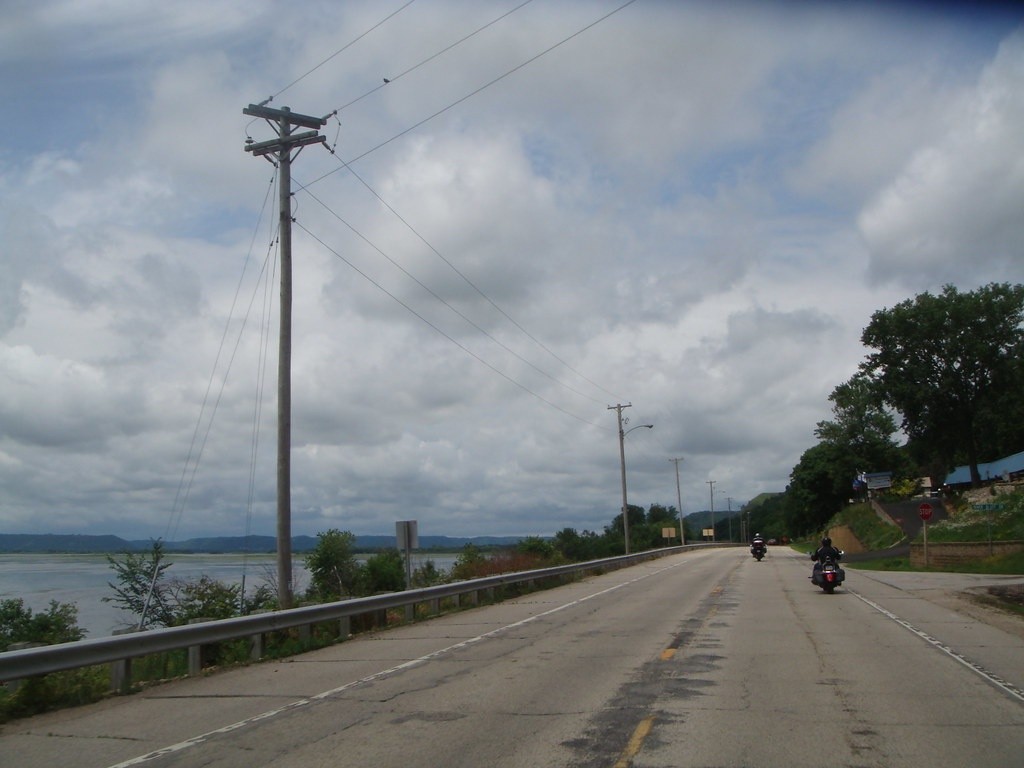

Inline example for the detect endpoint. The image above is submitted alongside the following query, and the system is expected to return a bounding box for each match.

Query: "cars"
[768,539,776,545]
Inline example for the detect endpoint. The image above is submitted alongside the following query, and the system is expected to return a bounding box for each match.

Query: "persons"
[751,533,767,555]
[811,538,845,583]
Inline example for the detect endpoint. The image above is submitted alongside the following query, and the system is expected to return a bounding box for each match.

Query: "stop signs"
[919,503,934,520]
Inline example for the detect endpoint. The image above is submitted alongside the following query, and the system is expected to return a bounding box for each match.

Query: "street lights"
[619,424,654,553]
[711,490,726,542]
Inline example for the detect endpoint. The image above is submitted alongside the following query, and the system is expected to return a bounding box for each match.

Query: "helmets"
[822,537,831,546]
[755,533,760,537]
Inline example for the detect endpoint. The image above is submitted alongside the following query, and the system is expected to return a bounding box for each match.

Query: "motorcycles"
[807,550,845,593]
[750,536,768,561]
